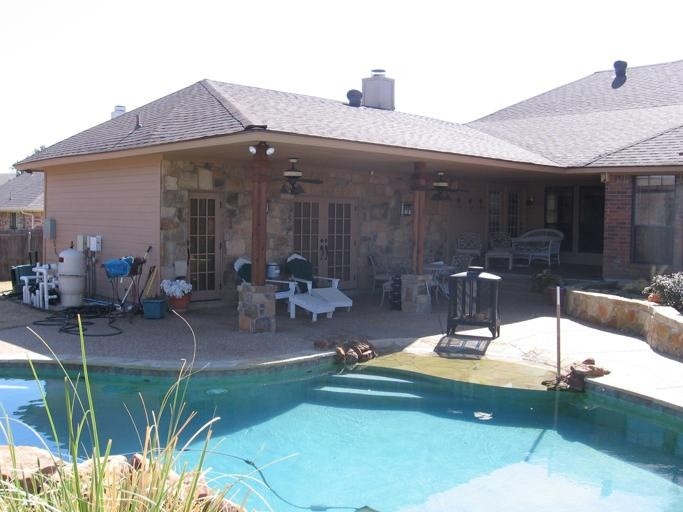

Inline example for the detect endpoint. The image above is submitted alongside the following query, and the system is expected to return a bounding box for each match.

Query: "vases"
[168,294,191,314]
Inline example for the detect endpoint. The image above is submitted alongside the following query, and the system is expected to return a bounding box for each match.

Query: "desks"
[424,264,457,303]
[484,249,533,270]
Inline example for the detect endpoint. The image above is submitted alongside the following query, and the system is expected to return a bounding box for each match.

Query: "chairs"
[367,255,390,294]
[442,255,473,300]
[234,252,341,313]
[455,231,482,264]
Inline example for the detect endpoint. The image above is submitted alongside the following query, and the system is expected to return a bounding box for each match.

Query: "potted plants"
[527,268,568,306]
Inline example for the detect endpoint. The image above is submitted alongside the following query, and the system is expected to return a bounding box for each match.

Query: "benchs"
[510,228,564,267]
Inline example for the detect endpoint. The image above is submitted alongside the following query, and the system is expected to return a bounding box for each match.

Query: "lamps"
[431,189,451,201]
[526,196,535,206]
[248,141,276,157]
[280,180,306,199]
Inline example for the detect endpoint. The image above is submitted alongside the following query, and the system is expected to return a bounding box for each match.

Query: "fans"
[430,171,465,192]
[269,158,323,184]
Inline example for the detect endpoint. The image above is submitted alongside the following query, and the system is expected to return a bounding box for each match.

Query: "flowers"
[159,278,193,300]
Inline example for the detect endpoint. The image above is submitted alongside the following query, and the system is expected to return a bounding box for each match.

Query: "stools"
[289,287,353,322]
